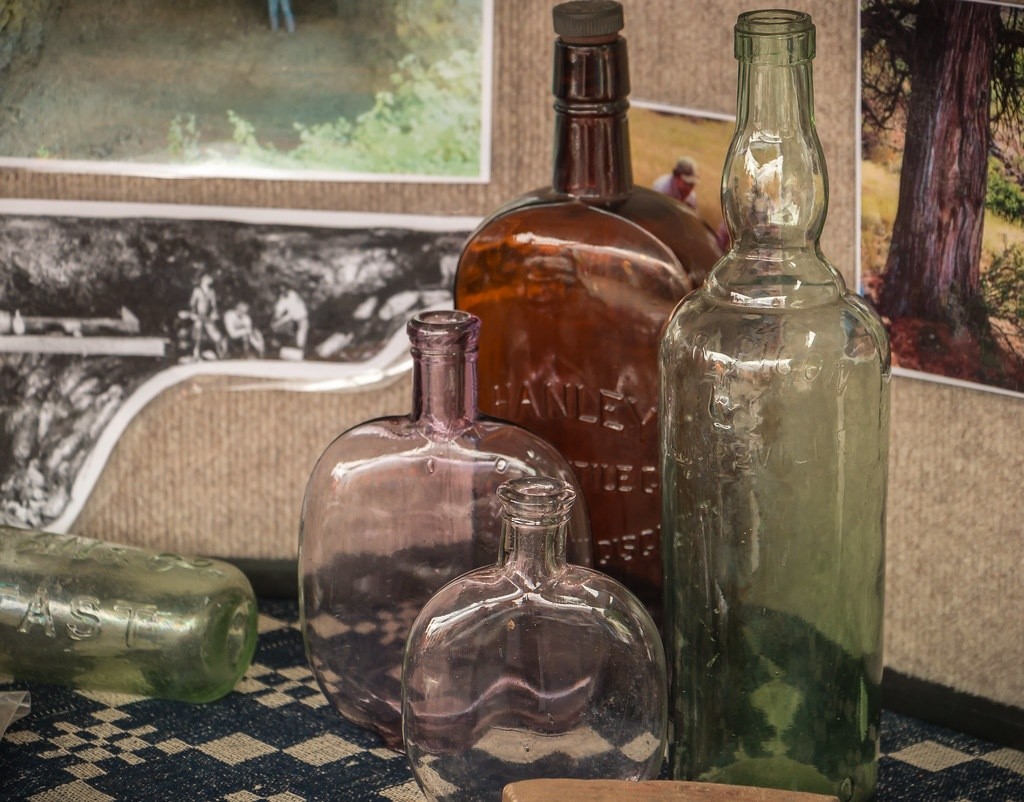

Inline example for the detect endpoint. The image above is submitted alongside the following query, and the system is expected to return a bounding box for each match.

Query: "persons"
[186,270,310,361]
[267,0,296,35]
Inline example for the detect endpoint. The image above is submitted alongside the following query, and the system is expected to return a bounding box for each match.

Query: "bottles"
[453,2,723,621]
[300,310,591,733]
[0,525,260,705]
[400,474,667,802]
[661,10,890,801]
